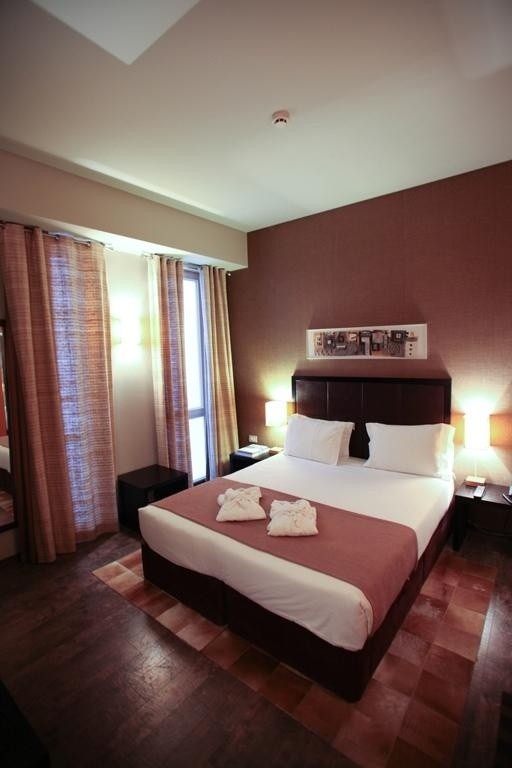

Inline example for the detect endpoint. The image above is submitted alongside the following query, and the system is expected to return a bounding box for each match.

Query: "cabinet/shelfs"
[116,462,187,541]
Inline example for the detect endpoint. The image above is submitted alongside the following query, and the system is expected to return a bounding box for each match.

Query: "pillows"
[284,412,346,465]
[337,418,356,460]
[360,421,456,482]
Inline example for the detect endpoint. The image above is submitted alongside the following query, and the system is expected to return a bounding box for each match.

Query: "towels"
[261,498,320,540]
[211,486,267,524]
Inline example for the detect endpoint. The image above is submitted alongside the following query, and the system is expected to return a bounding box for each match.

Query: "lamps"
[446,410,466,448]
[264,399,290,428]
[489,412,512,449]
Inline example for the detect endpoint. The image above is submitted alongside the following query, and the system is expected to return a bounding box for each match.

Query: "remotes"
[473,485,486,498]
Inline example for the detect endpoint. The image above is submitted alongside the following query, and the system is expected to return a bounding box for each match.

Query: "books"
[234,442,271,460]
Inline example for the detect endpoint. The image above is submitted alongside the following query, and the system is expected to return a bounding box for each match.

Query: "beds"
[134,371,461,707]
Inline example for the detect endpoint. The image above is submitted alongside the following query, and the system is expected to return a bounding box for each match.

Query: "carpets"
[91,527,505,765]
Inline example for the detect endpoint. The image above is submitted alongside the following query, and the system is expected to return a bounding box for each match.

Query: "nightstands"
[452,480,512,557]
[227,444,283,477]
